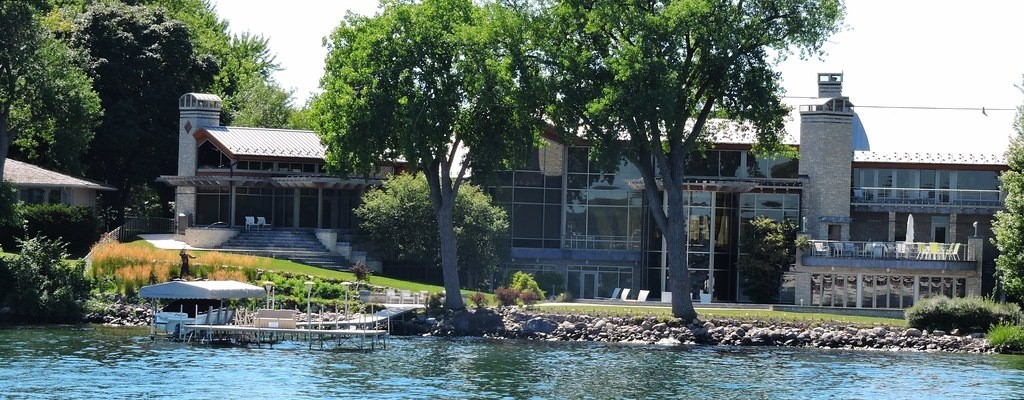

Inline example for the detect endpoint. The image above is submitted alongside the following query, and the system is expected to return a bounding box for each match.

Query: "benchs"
[253,307,298,329]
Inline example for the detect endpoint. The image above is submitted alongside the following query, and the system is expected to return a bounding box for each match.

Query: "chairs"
[915,191,929,204]
[830,241,961,261]
[416,291,429,304]
[257,217,271,231]
[386,289,400,304]
[853,190,868,203]
[245,217,259,231]
[815,243,830,258]
[400,290,416,304]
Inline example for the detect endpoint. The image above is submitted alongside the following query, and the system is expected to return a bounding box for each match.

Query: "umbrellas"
[905,213,914,243]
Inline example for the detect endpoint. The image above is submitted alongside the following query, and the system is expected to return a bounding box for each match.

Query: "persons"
[177,249,196,280]
